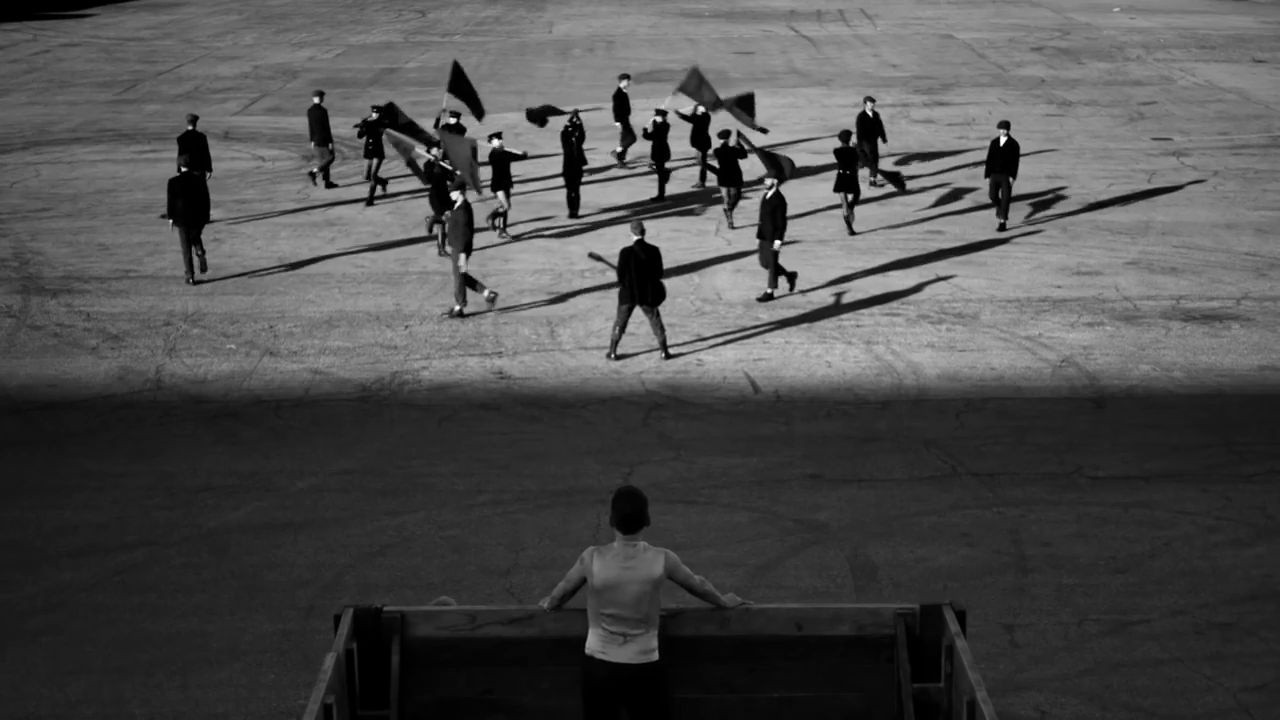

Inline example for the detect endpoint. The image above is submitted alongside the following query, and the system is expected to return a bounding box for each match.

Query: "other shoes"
[486,291,498,311]
[605,351,619,361]
[442,308,463,318]
[309,149,1010,257]
[185,277,195,285]
[788,271,798,292]
[199,252,207,273]
[755,289,774,302]
[661,352,672,360]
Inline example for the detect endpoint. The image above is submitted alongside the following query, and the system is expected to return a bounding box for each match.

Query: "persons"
[752,171,800,301]
[714,129,747,228]
[830,129,861,237]
[673,102,720,190]
[605,219,674,361]
[441,182,498,319]
[559,108,588,220]
[643,108,673,203]
[984,120,1021,233]
[484,130,530,239]
[354,104,388,208]
[540,486,756,717]
[611,72,638,168]
[158,113,214,220]
[423,141,456,258]
[856,96,888,188]
[167,155,212,286]
[307,88,340,189]
[432,109,468,161]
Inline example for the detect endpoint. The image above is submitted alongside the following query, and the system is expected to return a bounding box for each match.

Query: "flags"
[436,128,485,196]
[443,58,487,123]
[383,129,424,182]
[524,103,566,127]
[722,91,770,134]
[676,66,724,115]
[733,128,798,183]
[380,100,434,150]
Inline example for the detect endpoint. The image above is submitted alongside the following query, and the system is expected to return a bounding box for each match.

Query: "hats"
[186,113,199,121]
[996,120,1012,131]
[488,130,503,140]
[176,155,192,168]
[839,129,852,143]
[718,130,730,138]
[764,171,779,181]
[426,140,441,154]
[370,103,383,114]
[447,179,466,191]
[655,106,668,117]
[863,96,875,104]
[312,88,326,97]
[448,110,462,117]
